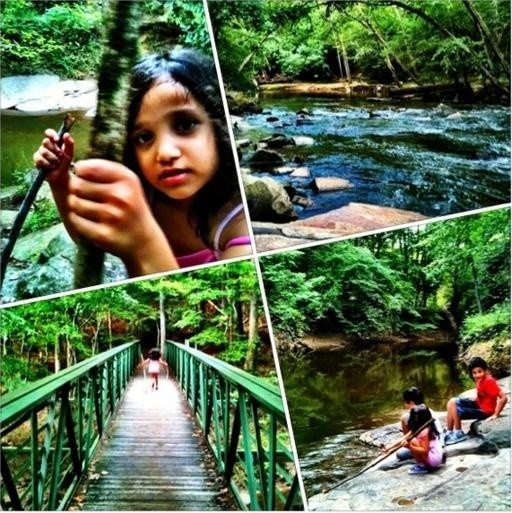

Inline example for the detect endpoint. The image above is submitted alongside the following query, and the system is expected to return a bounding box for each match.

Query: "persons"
[444,357,509,446]
[31,49,252,278]
[394,385,445,460]
[381,404,444,475]
[133,346,170,390]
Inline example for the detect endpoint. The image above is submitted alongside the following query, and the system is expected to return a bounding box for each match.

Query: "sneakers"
[408,464,428,474]
[441,429,468,445]
